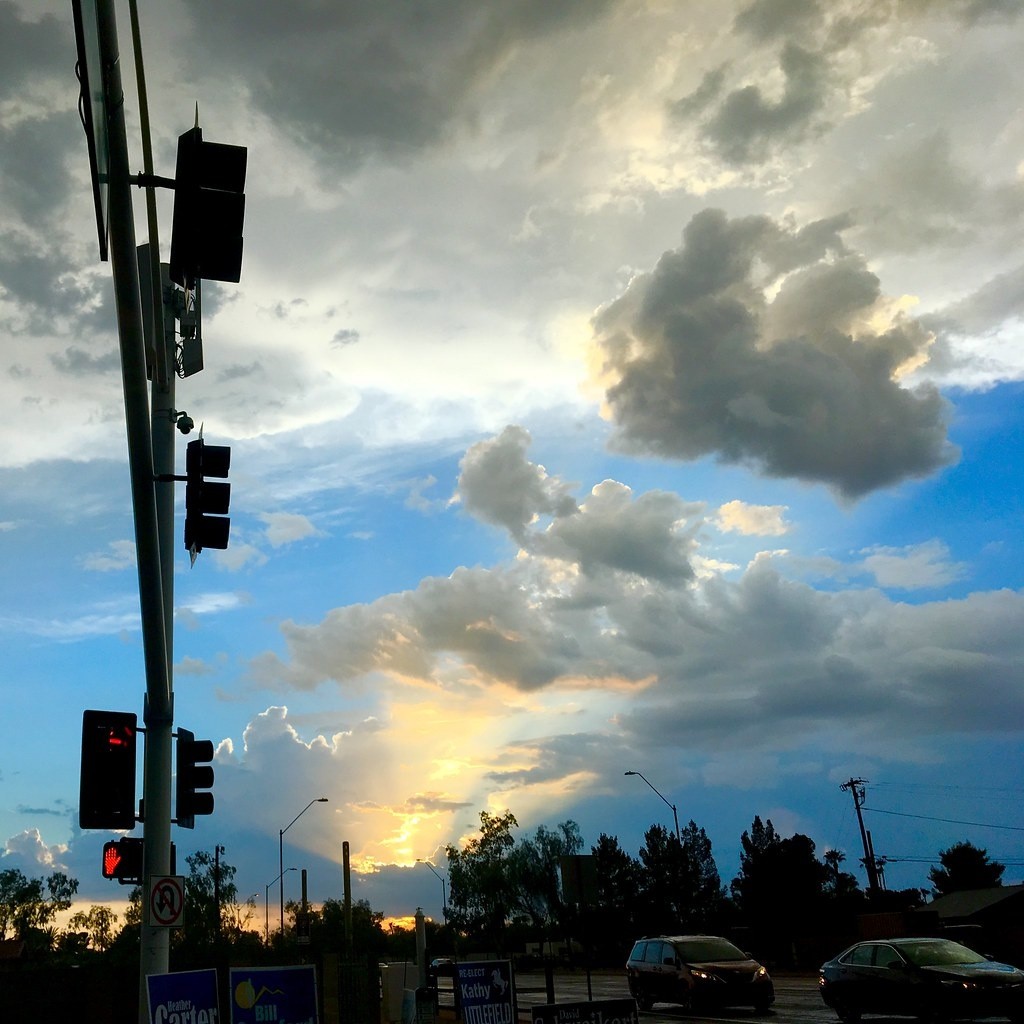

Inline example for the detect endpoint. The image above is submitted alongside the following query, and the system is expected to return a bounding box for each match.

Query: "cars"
[429,959,455,975]
[819,937,1024,1024]
[378,963,388,971]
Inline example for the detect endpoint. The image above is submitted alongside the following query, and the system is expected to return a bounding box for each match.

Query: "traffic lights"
[103,841,142,878]
[184,438,232,552]
[169,127,246,285]
[80,709,136,831]
[176,728,215,829]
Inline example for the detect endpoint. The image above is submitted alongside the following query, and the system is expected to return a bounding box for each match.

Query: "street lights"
[237,893,259,928]
[280,798,327,937]
[266,867,297,947]
[417,858,446,924]
[625,771,680,844]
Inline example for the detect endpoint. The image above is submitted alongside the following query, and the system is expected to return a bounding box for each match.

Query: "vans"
[626,935,775,1016]
